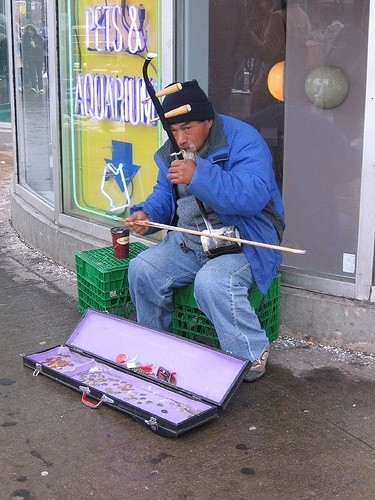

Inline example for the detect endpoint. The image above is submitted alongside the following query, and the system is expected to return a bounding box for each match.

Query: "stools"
[172,274,280,351]
[74,241,150,319]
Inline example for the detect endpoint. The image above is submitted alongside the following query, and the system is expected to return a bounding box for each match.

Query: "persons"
[123,78,286,383]
[22,24,44,93]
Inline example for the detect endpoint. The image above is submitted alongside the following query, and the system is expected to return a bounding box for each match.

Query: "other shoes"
[40,89,44,93]
[244,348,269,382]
[30,88,37,94]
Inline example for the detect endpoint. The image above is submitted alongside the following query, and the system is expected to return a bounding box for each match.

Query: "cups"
[110,226,131,258]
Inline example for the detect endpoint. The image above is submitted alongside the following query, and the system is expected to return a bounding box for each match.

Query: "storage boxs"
[20,308,253,437]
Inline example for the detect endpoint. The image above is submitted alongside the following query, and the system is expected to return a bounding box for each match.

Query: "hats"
[162,79,217,124]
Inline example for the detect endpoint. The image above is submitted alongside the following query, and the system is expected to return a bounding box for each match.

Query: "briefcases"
[21,308,253,438]
